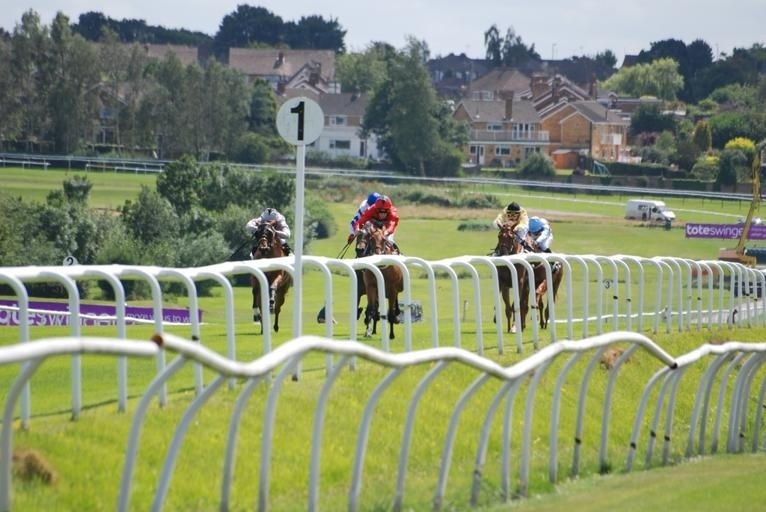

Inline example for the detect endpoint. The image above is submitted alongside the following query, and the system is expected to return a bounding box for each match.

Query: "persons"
[353,195,399,256]
[526,213,561,296]
[242,208,295,284]
[492,201,543,270]
[349,191,382,235]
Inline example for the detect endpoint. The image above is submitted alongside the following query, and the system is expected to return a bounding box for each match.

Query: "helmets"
[373,195,393,210]
[368,193,380,205]
[506,203,520,213]
[262,208,279,221]
[529,217,544,232]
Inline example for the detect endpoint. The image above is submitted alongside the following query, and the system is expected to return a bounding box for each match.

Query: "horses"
[492,219,564,334]
[249,219,293,333]
[355,220,405,340]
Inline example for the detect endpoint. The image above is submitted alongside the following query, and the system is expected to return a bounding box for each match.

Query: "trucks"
[624,199,678,223]
